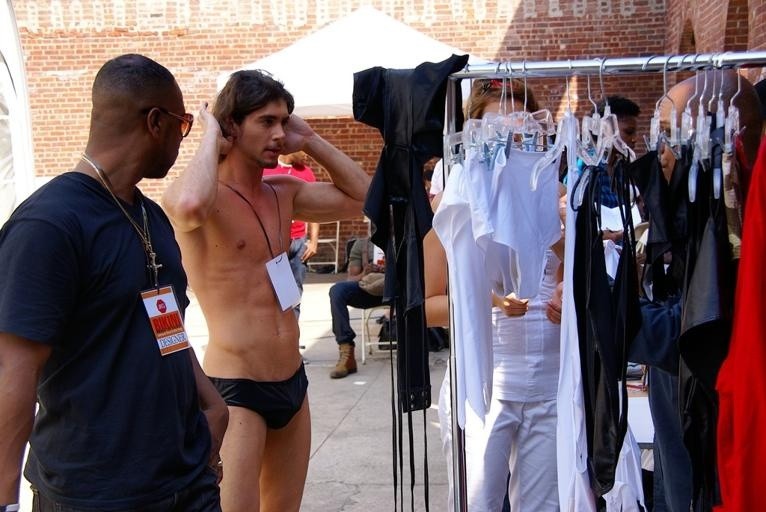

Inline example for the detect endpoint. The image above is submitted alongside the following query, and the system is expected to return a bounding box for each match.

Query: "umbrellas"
[222,8,488,120]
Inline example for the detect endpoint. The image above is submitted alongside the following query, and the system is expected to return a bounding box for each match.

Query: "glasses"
[142,105,194,137]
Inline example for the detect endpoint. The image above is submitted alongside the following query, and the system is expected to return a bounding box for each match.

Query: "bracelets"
[0,504,19,511]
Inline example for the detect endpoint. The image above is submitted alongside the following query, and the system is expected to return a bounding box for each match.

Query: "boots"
[330,344,358,378]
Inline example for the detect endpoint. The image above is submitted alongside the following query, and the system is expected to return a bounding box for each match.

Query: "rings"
[217,461,222,466]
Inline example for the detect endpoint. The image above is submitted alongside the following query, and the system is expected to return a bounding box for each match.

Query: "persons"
[264,149,319,366]
[565,94,641,294]
[161,70,370,511]
[0,54,230,511]
[640,69,761,178]
[328,209,388,379]
[422,78,568,512]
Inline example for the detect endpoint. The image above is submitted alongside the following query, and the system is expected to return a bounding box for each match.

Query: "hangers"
[463,62,555,159]
[574,58,638,170]
[642,51,742,160]
[528,59,580,192]
[443,64,473,166]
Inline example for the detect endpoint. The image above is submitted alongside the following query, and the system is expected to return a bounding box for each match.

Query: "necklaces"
[80,151,164,288]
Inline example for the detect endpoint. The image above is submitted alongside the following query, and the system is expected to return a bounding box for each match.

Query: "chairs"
[306,220,339,274]
[362,307,398,366]
